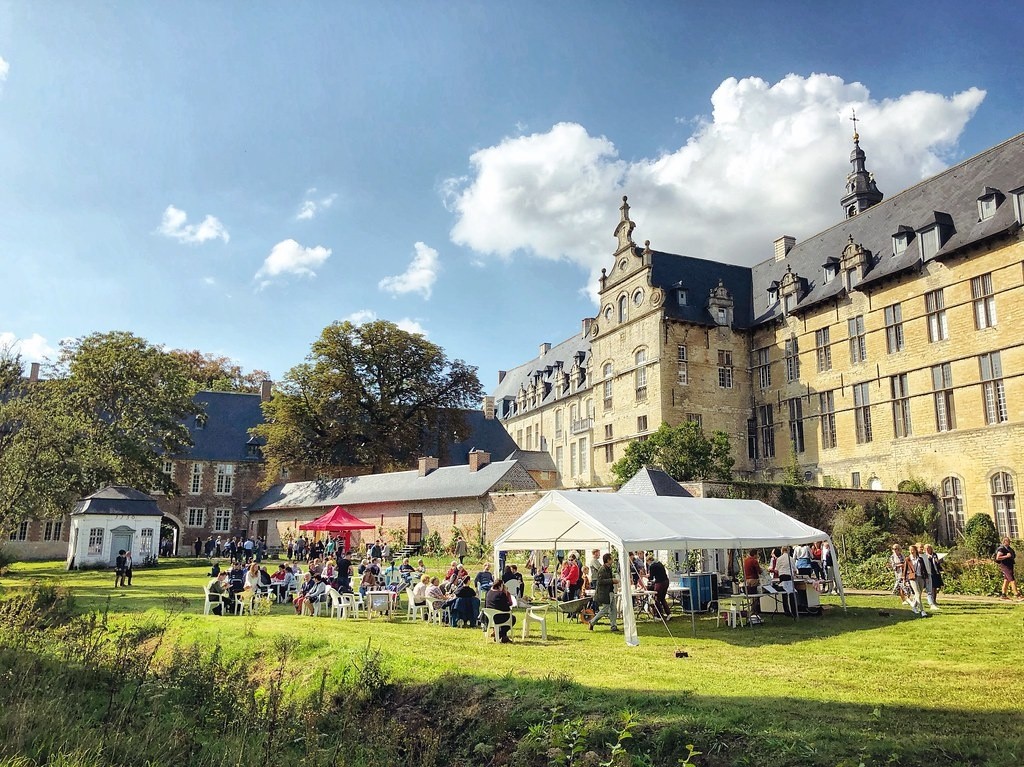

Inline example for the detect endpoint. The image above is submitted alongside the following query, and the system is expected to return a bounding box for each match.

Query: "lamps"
[805,471,813,480]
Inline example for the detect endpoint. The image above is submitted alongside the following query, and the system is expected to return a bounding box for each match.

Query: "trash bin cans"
[681,571,718,614]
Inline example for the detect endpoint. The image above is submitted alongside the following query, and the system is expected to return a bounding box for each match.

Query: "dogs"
[515,595,534,608]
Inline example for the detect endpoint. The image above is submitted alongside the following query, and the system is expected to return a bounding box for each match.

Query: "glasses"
[569,559,575,561]
[891,548,898,550]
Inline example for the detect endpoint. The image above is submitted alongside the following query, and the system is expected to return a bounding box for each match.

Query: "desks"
[717,593,745,628]
[616,591,658,619]
[730,592,793,625]
[669,587,689,612]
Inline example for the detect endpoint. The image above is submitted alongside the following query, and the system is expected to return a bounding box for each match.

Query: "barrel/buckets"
[732,578,740,595]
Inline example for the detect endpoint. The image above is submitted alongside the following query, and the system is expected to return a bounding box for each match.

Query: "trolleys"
[547,597,592,624]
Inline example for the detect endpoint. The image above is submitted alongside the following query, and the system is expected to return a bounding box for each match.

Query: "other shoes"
[589,621,593,630]
[913,608,919,614]
[1001,594,1010,600]
[501,636,512,643]
[906,598,912,604]
[902,601,908,604]
[930,605,941,610]
[1016,593,1024,601]
[667,614,672,620]
[611,625,619,631]
[921,611,927,616]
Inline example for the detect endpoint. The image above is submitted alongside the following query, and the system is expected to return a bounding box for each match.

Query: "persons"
[286,532,344,564]
[349,540,392,566]
[498,551,508,576]
[528,550,620,620]
[205,536,269,566]
[588,553,621,632]
[114,550,132,588]
[161,536,173,558]
[209,555,524,643]
[629,550,644,589]
[194,537,202,558]
[995,537,1024,599]
[768,541,839,595]
[889,542,947,617]
[640,555,672,621]
[454,536,467,564]
[775,546,797,616]
[744,548,764,615]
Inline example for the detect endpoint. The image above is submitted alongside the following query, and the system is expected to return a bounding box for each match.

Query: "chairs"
[559,597,592,624]
[387,570,518,644]
[204,574,365,619]
[522,603,550,639]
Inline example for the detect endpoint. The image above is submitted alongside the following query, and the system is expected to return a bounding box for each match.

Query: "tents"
[492,489,847,648]
[299,506,376,546]
